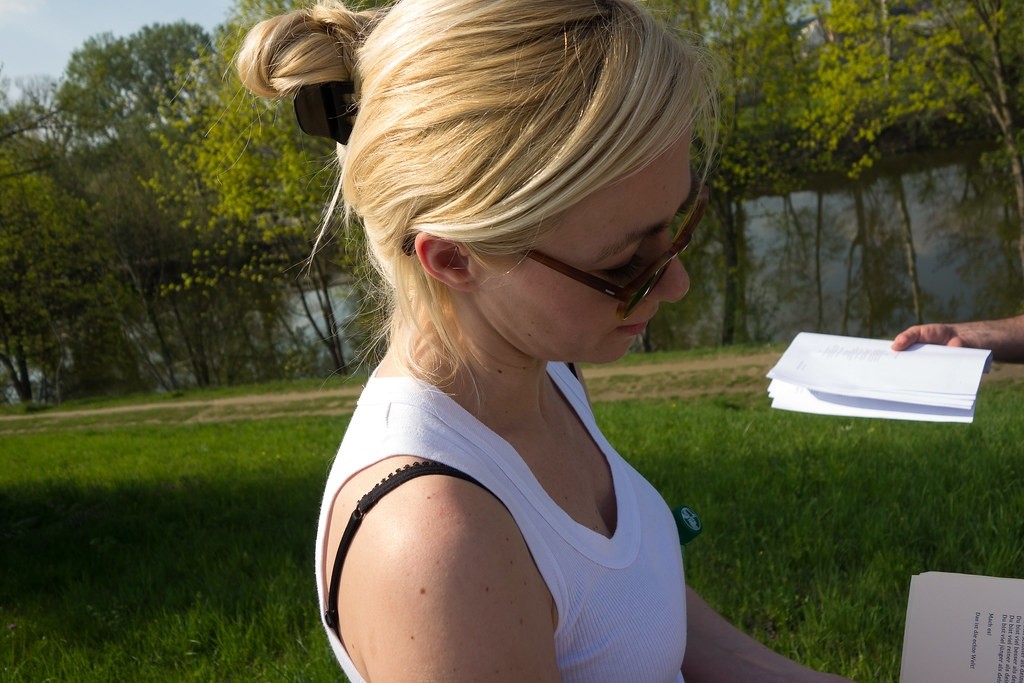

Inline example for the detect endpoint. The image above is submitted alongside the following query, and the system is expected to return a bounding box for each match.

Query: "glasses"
[403,178,708,317]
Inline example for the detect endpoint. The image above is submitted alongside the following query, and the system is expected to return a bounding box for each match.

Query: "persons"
[240,2,849,682]
[890,309,1024,368]
